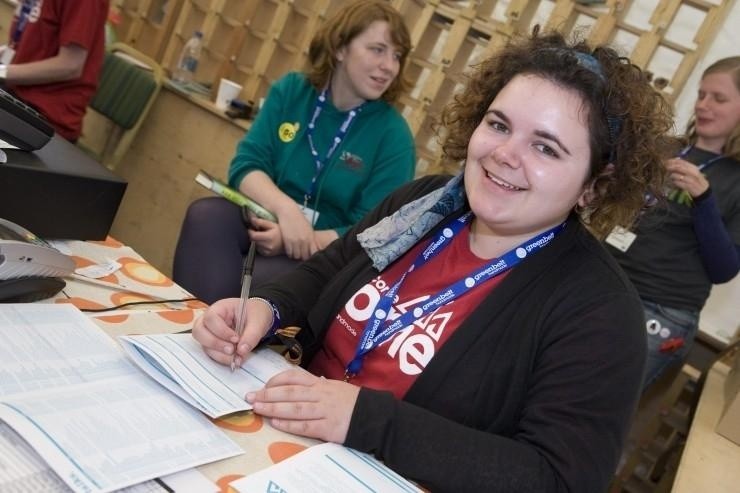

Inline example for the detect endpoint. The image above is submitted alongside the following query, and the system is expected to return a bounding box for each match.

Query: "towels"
[355,174,471,274]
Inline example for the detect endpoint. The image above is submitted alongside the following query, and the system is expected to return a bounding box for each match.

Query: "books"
[194,169,277,223]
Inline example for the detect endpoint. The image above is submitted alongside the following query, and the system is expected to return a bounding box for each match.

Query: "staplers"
[225,99,254,118]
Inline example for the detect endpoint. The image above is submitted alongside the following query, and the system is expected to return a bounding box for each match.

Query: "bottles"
[171,30,203,84]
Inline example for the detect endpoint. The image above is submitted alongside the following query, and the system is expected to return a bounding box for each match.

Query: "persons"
[0,0,110,144]
[600,57,739,393]
[174,0,416,306]
[192,25,689,493]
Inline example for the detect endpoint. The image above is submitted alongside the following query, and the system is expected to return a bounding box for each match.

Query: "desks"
[0,231,433,493]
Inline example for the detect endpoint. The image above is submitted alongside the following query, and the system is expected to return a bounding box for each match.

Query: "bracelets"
[0,64,7,79]
[249,296,280,341]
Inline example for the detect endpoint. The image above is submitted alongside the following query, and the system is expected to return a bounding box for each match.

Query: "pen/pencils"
[230,240,258,373]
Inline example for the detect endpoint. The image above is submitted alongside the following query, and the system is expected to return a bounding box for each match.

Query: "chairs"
[76,42,166,172]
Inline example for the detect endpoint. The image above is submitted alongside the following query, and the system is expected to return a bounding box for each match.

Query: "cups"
[214,78,242,110]
